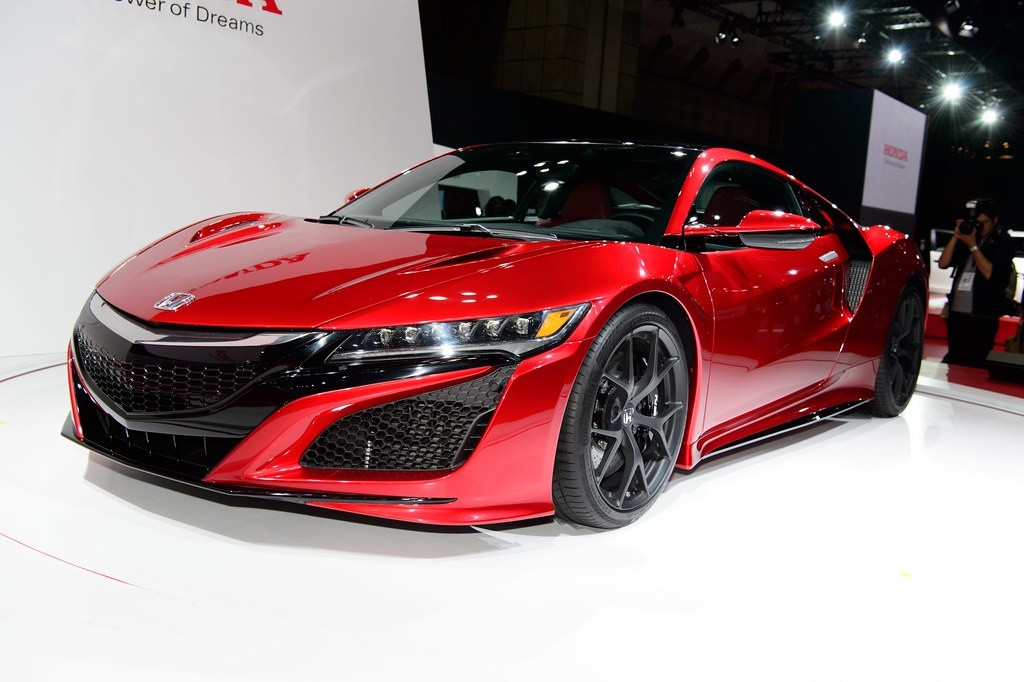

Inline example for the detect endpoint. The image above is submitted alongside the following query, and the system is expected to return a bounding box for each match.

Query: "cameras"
[958,199,983,236]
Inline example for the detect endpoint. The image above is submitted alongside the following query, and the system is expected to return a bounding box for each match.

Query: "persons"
[936,203,1018,366]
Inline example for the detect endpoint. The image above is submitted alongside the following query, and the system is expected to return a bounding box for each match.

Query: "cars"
[59,137,932,535]
